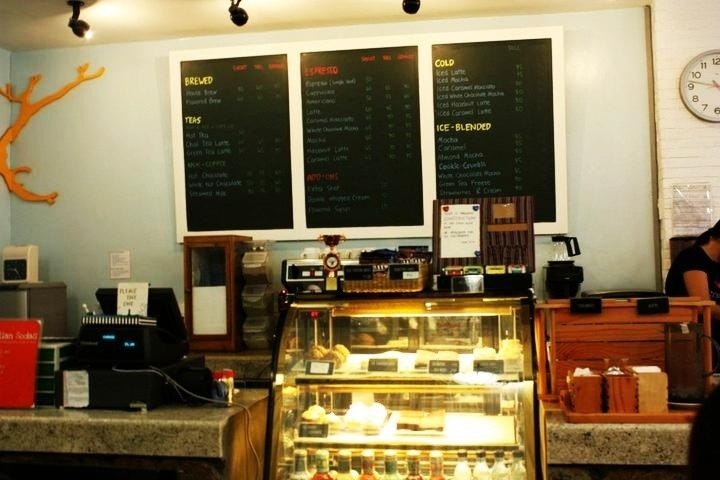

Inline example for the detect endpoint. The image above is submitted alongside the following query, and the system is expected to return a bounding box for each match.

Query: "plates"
[668,401,704,410]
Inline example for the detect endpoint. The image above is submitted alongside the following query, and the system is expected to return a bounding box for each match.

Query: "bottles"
[212,370,234,408]
[286,448,527,480]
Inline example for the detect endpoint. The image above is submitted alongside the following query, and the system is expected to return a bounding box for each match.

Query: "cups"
[301,247,366,260]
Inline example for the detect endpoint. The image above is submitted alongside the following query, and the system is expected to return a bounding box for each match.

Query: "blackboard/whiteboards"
[169,24,569,244]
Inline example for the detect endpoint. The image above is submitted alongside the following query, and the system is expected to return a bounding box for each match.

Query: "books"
[81,316,158,327]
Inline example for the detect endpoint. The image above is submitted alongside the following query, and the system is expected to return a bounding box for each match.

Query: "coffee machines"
[274,258,359,344]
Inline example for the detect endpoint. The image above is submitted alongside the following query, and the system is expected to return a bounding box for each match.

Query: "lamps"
[228,0,249,26]
[62,0,90,38]
[401,0,422,13]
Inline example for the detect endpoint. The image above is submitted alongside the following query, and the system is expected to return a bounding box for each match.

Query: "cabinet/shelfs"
[181,236,257,352]
[266,291,540,480]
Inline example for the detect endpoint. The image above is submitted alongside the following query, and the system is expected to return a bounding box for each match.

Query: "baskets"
[341,265,430,295]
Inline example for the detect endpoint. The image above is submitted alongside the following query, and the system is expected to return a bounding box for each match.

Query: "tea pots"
[545,234,582,266]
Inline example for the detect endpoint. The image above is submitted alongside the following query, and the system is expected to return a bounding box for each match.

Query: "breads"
[305,342,352,371]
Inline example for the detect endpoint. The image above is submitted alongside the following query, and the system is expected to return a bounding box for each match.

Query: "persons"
[664,218,720,376]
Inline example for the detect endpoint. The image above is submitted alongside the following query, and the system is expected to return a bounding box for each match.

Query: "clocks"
[678,50,720,124]
[1,243,41,284]
